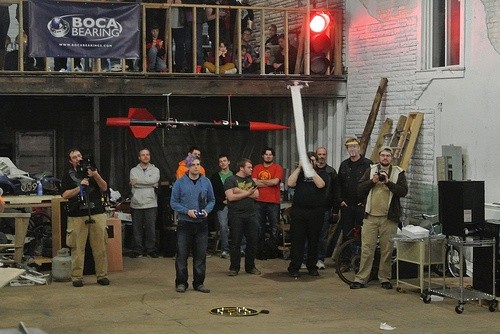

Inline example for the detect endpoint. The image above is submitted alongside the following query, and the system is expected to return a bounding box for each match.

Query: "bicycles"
[3,207,52,250]
[333,214,467,286]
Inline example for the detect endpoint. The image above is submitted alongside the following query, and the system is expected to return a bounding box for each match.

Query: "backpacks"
[0,175,38,195]
[32,170,64,195]
[26,222,52,257]
[82,248,95,276]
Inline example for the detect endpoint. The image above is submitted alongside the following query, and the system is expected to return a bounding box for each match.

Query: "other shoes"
[309,268,319,276]
[149,251,159,258]
[250,266,261,274]
[59,68,67,73]
[289,270,300,276]
[73,280,83,287]
[316,259,326,269]
[241,250,245,256]
[300,263,307,268]
[230,68,237,74]
[354,266,359,273]
[350,282,365,289]
[381,282,392,289]
[336,264,350,272]
[97,278,109,285]
[221,251,229,257]
[196,284,210,293]
[176,284,186,292]
[205,68,210,73]
[111,63,129,71]
[228,270,238,276]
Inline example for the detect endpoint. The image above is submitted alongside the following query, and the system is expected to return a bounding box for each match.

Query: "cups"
[196,66,202,73]
[431,295,443,302]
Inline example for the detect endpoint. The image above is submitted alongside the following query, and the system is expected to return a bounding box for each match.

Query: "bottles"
[202,22,209,35]
[38,183,42,196]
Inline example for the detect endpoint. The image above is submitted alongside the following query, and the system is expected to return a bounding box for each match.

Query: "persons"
[222,158,261,276]
[0,0,297,74]
[60,148,110,286]
[348,145,408,291]
[175,147,206,180]
[170,156,215,293]
[287,151,328,277]
[334,138,373,273]
[250,147,284,259]
[129,146,160,258]
[210,155,247,258]
[299,147,338,269]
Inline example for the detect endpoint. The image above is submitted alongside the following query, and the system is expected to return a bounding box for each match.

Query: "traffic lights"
[308,10,330,74]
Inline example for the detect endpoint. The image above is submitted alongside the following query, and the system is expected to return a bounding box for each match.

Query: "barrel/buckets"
[270,45,279,55]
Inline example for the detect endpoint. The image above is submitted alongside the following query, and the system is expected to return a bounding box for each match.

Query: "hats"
[378,146,393,155]
[346,139,358,145]
[185,155,198,168]
[307,151,318,161]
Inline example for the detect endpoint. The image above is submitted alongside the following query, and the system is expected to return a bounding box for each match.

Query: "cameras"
[375,171,387,182]
